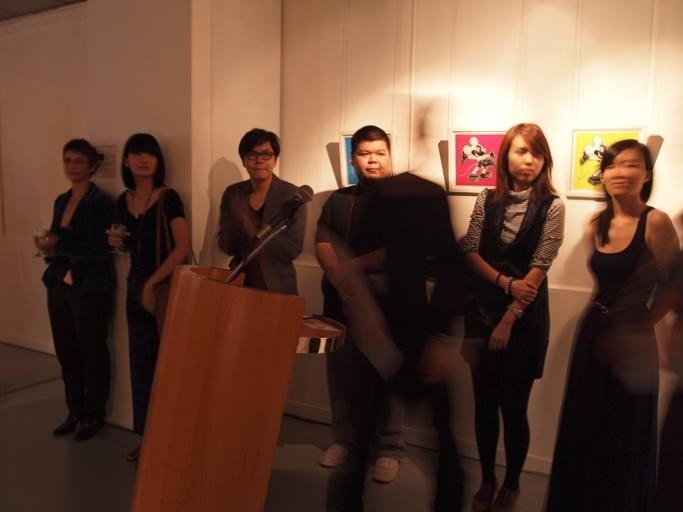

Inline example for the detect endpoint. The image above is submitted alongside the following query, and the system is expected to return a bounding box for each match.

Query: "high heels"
[75,422,103,441]
[472,480,498,512]
[493,483,519,512]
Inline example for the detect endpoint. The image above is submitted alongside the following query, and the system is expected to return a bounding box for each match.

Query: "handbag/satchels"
[153,188,198,340]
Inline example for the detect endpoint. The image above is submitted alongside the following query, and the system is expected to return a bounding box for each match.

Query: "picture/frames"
[340,133,394,188]
[447,129,508,193]
[564,129,643,200]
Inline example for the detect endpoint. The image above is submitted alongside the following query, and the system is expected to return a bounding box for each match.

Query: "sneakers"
[374,456,399,482]
[320,444,348,467]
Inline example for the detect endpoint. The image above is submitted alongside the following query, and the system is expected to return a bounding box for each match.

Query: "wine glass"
[32,228,48,257]
[108,223,127,255]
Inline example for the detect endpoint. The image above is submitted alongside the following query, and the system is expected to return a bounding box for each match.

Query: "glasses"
[247,150,274,160]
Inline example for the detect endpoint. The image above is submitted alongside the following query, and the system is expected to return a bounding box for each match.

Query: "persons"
[36,138,115,442]
[105,133,190,469]
[312,124,428,485]
[462,122,565,512]
[217,125,306,296]
[546,140,681,511]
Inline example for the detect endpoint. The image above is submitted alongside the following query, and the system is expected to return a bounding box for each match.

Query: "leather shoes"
[53,418,78,436]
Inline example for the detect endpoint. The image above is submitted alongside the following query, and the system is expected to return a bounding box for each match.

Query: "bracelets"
[507,304,525,318]
[504,274,516,295]
[493,270,503,287]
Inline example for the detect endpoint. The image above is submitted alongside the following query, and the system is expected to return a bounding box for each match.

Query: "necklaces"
[129,184,156,220]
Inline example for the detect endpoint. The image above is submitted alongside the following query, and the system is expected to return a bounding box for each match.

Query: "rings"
[518,297,523,302]
[45,237,51,242]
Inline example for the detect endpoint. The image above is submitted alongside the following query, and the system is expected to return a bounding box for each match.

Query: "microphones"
[251,185,313,243]
